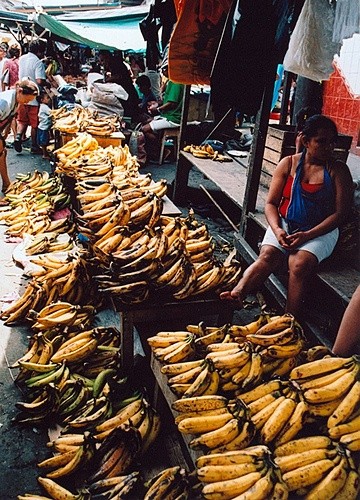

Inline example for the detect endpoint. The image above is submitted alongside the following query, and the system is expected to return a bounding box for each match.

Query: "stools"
[159,128,179,164]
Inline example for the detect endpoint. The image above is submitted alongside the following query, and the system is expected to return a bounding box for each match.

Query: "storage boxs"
[259,125,353,191]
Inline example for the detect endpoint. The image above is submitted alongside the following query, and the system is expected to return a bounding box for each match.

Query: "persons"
[0,79,39,192]
[135,74,157,124]
[219,115,354,332]
[14,40,43,155]
[331,283,360,357]
[141,43,185,160]
[1,47,20,142]
[44,45,96,79]
[113,48,144,84]
[141,60,162,101]
[0,42,9,73]
[9,96,30,140]
[36,88,52,158]
[98,50,139,123]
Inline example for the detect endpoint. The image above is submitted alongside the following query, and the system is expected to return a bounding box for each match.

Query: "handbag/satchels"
[129,122,147,164]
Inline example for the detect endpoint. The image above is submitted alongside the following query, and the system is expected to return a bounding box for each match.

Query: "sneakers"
[14,140,22,152]
[32,148,42,154]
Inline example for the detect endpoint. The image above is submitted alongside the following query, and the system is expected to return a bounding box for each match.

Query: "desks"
[111,287,236,374]
[174,149,283,237]
[59,131,125,152]
[139,173,182,216]
[151,337,264,498]
[231,207,360,357]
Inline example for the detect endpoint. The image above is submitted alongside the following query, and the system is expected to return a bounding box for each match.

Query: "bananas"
[160,357,219,399]
[274,437,359,500]
[18,476,92,500]
[171,395,256,454]
[1,255,106,326]
[229,305,305,381]
[196,445,288,500]
[52,133,243,305]
[236,379,307,446]
[204,342,264,396]
[146,320,233,364]
[89,470,145,500]
[144,465,203,500]
[38,431,96,478]
[0,169,76,256]
[288,353,360,454]
[10,301,163,433]
[92,421,141,480]
